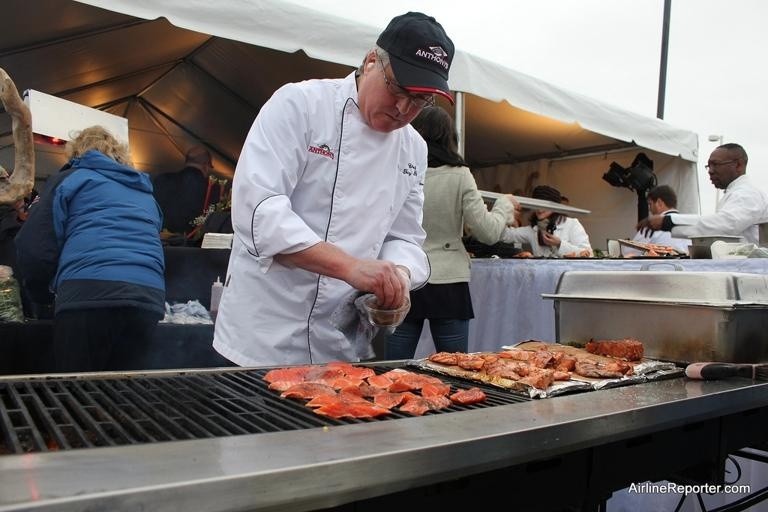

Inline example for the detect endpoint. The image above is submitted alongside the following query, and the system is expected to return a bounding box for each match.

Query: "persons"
[206,12,458,369]
[46,137,166,369]
[150,144,222,235]
[382,104,520,359]
[501,182,594,258]
[619,183,695,258]
[13,124,116,322]
[461,219,521,259]
[635,142,768,246]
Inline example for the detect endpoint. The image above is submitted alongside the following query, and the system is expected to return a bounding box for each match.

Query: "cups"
[705,159,738,170]
[376,53,435,108]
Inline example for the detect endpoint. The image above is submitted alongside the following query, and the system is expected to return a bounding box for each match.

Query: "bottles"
[532,185,562,204]
[376,11,456,107]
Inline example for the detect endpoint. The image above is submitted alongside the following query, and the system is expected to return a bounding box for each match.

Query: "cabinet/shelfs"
[209,275,223,317]
[0,265,25,322]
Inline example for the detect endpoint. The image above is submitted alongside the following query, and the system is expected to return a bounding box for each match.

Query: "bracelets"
[365,293,410,326]
